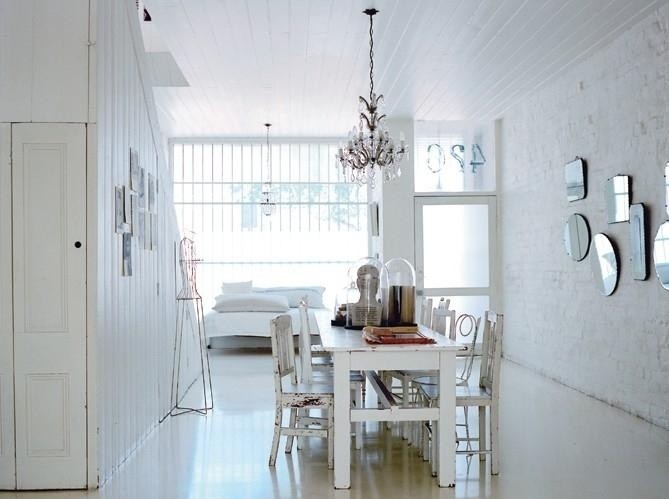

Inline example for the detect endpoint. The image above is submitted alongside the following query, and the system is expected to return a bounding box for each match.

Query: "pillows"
[211,280,325,312]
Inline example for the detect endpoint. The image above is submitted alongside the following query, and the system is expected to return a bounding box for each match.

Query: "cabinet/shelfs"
[9,124,86,492]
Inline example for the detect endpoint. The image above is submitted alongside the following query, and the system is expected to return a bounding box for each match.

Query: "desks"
[315,310,469,489]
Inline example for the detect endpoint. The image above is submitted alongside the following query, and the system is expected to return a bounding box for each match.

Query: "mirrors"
[563,156,585,202]
[588,232,618,296]
[653,221,669,291]
[563,213,590,261]
[607,175,629,224]
[663,161,668,216]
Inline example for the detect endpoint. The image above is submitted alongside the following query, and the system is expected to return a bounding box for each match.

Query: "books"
[362,326,434,345]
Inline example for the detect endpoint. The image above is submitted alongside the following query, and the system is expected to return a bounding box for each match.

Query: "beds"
[200,286,335,351]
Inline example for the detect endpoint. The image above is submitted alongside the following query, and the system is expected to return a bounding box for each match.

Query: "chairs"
[419,310,502,478]
[297,306,365,451]
[296,300,361,372]
[377,297,480,457]
[270,314,335,471]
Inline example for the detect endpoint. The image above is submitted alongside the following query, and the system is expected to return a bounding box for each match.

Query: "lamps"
[335,8,410,190]
[259,123,276,217]
